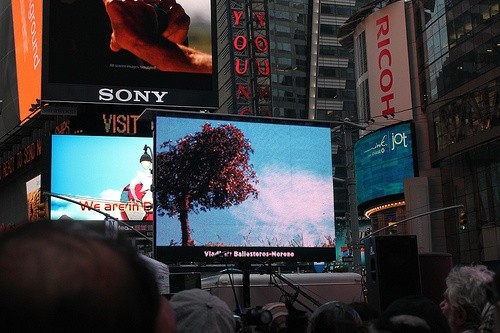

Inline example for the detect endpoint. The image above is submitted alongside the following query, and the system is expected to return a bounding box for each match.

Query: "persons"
[104,0,212,73]
[0,217,500,333]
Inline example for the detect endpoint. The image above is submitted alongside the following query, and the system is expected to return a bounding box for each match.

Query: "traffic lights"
[37,201,50,221]
[458,211,468,231]
